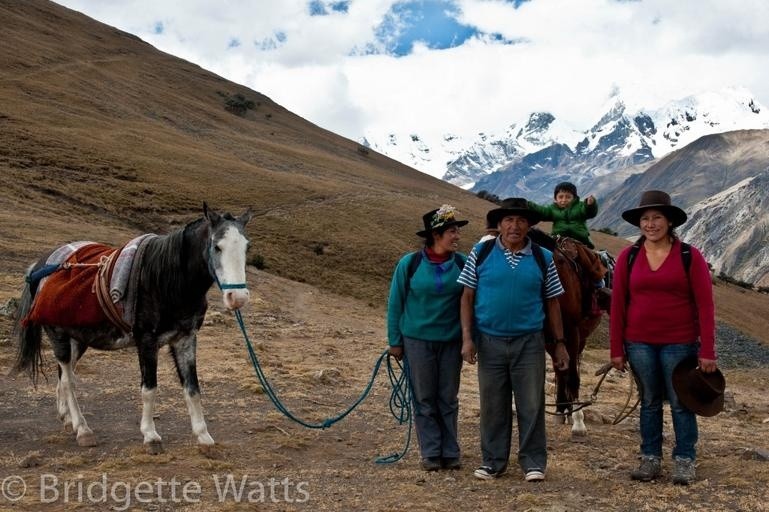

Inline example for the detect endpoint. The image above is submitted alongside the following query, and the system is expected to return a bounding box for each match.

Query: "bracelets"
[553,338,567,344]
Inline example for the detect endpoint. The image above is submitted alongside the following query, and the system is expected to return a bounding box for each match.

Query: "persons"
[454,197,570,481]
[524,181,599,251]
[610,189,717,486]
[386,203,480,471]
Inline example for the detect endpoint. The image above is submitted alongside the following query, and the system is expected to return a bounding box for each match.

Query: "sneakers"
[473,465,498,482]
[523,466,546,482]
[670,455,696,483]
[443,457,461,470]
[628,455,663,481]
[421,457,442,471]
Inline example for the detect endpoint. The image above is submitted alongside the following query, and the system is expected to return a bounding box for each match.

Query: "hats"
[411,208,470,237]
[672,361,727,416]
[623,190,692,228]
[489,194,543,230]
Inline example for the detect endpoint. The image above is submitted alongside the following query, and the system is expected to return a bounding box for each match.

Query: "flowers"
[432,202,457,225]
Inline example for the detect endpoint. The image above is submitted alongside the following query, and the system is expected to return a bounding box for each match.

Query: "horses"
[13,200,254,455]
[487,227,613,443]
